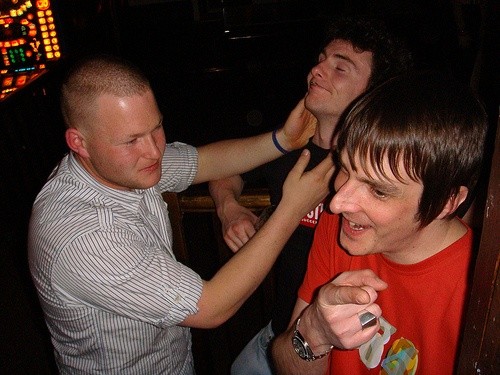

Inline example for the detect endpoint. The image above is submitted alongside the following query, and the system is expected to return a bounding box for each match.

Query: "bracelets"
[272,129,292,155]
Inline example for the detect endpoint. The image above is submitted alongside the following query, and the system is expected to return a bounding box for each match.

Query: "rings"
[357,309,377,330]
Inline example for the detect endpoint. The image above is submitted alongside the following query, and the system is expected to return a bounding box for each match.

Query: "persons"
[270,76,496,375]
[212,24,395,373]
[29,58,338,375]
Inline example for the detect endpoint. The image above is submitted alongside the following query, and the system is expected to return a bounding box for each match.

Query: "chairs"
[160,187,274,274]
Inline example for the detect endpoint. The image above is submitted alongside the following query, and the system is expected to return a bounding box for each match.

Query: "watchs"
[291,316,334,362]
